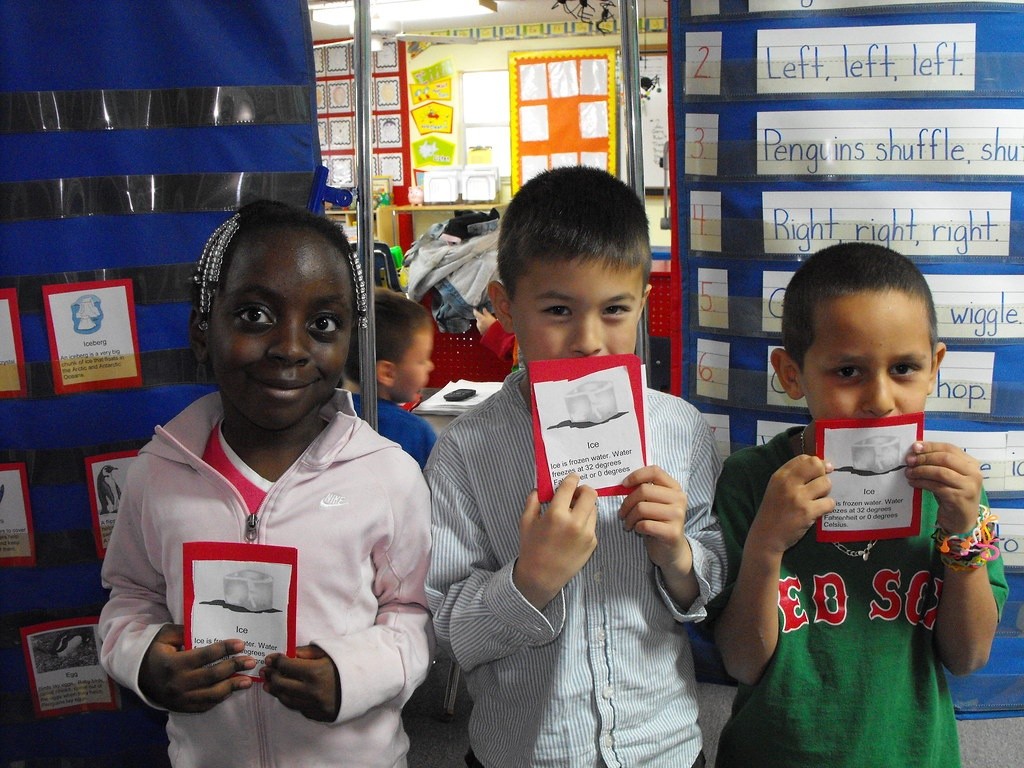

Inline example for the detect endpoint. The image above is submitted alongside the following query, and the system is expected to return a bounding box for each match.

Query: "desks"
[375,204,505,247]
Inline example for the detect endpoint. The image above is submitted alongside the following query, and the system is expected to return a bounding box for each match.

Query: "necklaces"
[799,431,879,562]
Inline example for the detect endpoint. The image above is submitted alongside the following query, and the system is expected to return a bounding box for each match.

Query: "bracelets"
[930,505,1003,573]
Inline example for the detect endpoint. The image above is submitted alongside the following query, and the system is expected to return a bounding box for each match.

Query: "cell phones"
[444,389,476,401]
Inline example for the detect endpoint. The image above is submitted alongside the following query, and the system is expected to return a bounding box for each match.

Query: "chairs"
[414,249,522,388]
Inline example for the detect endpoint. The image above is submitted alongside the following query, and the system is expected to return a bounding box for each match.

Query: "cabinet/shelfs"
[324,205,400,248]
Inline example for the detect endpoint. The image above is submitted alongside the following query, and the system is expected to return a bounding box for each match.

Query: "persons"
[341,289,519,470]
[97,200,435,768]
[691,242,1009,768]
[423,164,728,767]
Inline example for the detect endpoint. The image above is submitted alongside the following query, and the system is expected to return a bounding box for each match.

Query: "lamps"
[308,0,497,25]
